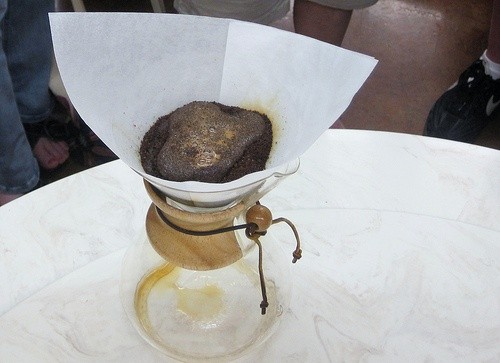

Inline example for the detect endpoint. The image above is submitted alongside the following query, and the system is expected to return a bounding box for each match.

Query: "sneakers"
[422,59,500,144]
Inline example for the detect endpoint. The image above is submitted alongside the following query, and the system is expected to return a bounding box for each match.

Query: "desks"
[0,130,500,363]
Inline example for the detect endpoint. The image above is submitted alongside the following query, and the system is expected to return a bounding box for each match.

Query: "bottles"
[126,177,285,363]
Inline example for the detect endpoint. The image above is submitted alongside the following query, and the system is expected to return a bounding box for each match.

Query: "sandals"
[23,88,75,179]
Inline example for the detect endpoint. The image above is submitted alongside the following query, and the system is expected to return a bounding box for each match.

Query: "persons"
[0,0,382,205]
[421,0,500,145]
